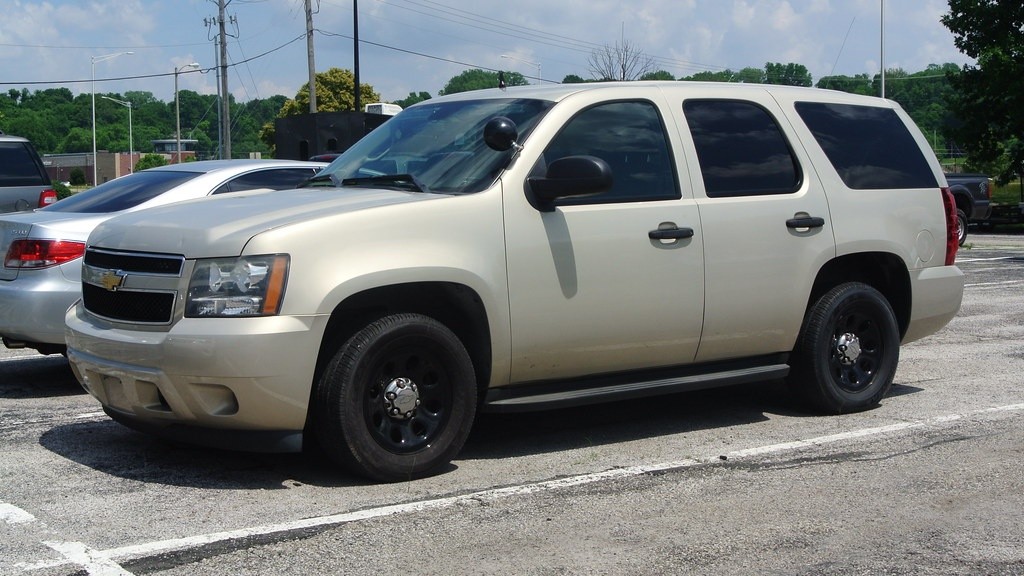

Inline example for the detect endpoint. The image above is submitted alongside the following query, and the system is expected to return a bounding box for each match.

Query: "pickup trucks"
[942,172,992,247]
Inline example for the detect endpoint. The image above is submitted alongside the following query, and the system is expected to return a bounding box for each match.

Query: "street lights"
[91,51,134,189]
[101,93,134,176]
[501,54,542,85]
[173,63,200,164]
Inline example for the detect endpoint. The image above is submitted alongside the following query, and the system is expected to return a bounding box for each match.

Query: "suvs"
[59,78,967,484]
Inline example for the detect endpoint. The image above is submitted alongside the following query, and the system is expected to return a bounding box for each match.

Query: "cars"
[0,133,57,213]
[0,156,406,359]
